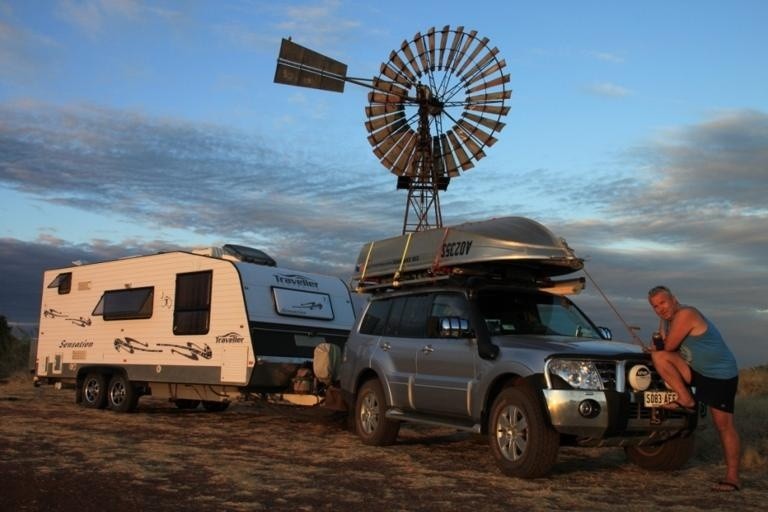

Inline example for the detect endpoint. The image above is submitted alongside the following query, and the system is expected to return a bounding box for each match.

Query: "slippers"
[662,401,696,413]
[711,481,743,491]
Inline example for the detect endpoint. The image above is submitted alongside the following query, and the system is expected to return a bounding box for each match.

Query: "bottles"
[651,331,664,352]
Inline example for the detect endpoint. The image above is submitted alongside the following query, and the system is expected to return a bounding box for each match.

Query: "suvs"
[328,214,706,475]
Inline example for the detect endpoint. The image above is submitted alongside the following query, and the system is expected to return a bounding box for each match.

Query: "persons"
[649,287,742,491]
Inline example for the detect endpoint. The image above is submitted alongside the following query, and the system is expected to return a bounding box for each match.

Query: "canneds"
[652,332,665,351]
